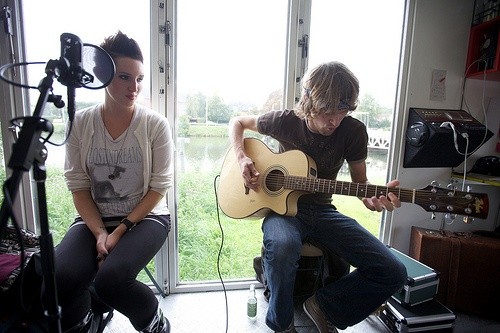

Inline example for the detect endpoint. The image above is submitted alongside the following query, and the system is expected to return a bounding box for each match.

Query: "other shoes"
[140,305,171,333]
[303,294,339,333]
[275,317,299,333]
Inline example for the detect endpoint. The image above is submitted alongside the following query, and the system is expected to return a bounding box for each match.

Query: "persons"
[53,30,173,333]
[229,60,408,333]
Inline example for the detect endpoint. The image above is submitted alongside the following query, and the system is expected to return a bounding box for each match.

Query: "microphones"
[60,33,83,121]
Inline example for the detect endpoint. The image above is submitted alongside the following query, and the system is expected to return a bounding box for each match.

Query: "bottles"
[247,284,257,320]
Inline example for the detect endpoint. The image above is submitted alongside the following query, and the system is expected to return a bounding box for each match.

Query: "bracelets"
[120,217,136,232]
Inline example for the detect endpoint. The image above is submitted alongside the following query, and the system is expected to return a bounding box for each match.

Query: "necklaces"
[103,104,136,180]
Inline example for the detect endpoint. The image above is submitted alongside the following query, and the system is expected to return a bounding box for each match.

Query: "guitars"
[218,137,489,225]
